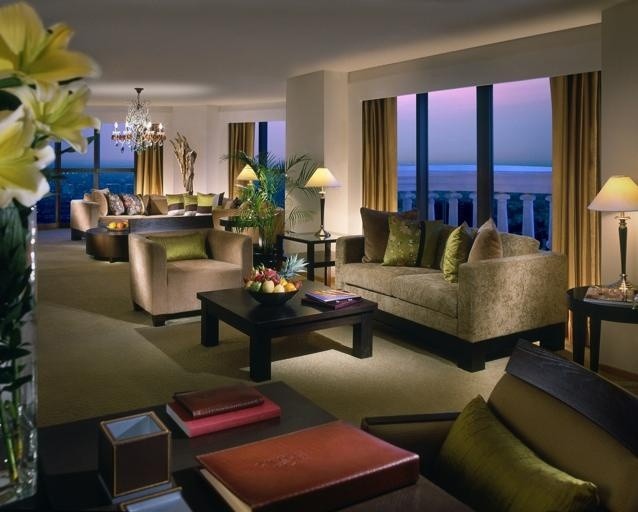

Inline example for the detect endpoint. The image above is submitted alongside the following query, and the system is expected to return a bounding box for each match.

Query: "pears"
[262,277,296,293]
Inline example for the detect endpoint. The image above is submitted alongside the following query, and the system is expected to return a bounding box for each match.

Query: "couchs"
[332,218,571,375]
[352,337,638,510]
[65,185,238,240]
[210,206,287,247]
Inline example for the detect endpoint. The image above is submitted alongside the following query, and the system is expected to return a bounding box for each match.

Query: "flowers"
[0,1,114,485]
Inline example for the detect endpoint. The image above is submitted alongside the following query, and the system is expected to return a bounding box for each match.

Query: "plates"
[103,225,129,232]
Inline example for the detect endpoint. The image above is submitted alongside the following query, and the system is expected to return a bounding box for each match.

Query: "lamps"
[108,85,166,154]
[303,166,343,238]
[235,162,259,190]
[587,172,637,298]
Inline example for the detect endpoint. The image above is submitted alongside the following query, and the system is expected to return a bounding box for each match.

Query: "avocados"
[249,280,262,294]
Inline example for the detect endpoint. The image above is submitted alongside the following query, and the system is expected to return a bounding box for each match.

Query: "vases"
[0,346,44,505]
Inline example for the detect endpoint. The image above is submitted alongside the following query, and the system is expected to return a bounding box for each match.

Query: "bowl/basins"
[248,286,299,309]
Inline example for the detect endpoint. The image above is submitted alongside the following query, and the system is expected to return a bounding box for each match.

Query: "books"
[303,287,363,304]
[301,294,366,311]
[195,416,421,510]
[163,386,282,438]
[171,381,264,421]
[583,283,638,309]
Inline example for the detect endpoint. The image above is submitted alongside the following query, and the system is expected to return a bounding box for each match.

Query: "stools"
[82,223,129,262]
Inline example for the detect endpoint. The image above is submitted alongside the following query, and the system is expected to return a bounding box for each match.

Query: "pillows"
[438,223,469,282]
[149,231,211,262]
[380,210,443,267]
[467,217,503,266]
[84,186,224,216]
[427,393,601,510]
[357,205,421,264]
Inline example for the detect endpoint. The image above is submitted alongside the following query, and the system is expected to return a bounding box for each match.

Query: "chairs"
[125,225,256,330]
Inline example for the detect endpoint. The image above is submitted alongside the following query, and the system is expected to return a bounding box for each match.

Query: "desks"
[193,277,379,382]
[275,229,352,284]
[561,281,638,374]
[1,374,476,512]
[218,216,284,273]
